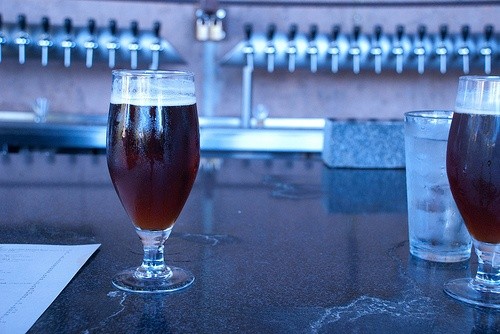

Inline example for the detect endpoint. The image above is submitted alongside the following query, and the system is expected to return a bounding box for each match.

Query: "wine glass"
[444,74,500,309]
[105,69,200,295]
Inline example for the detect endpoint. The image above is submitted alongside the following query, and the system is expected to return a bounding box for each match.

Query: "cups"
[403,109,473,266]
